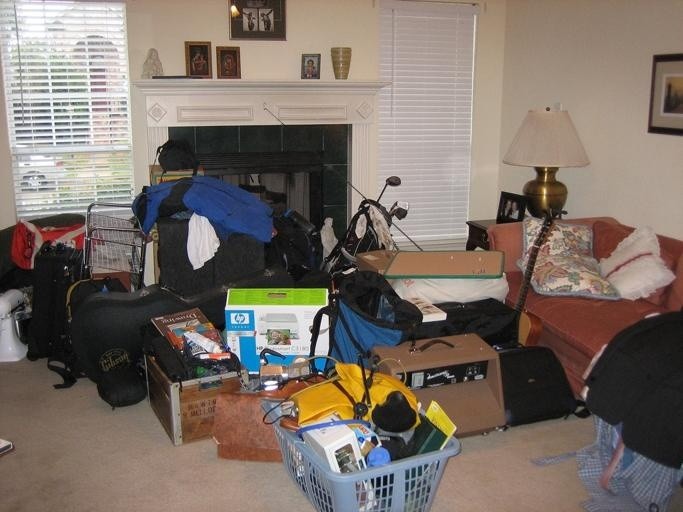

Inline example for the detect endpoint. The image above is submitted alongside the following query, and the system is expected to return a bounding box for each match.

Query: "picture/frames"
[301,53,321,79]
[185,41,212,79]
[497,191,527,225]
[229,0,286,41]
[648,53,683,136]
[216,46,241,79]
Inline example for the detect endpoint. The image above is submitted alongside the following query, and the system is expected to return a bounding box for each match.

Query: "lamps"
[503,107,589,218]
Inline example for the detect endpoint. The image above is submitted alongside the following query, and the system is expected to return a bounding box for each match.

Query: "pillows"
[517,255,620,300]
[598,226,676,301]
[523,218,593,258]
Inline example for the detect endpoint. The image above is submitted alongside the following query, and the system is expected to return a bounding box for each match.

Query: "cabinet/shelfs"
[134,80,392,238]
[466,220,497,250]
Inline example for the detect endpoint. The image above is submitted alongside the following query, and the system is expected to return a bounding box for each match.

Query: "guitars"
[516,203,569,347]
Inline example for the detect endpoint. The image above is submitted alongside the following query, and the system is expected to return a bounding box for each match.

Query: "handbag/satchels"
[150,140,199,186]
[214,392,288,463]
[496,345,591,435]
[407,298,521,348]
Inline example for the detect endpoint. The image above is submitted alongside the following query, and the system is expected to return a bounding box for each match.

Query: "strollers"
[312,176,409,298]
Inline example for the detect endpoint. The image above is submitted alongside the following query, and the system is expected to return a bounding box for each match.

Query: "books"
[405,298,447,322]
[149,164,204,186]
[151,308,231,360]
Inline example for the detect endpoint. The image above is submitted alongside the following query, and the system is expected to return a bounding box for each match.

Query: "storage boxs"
[260,397,460,512]
[145,354,248,446]
[225,289,330,375]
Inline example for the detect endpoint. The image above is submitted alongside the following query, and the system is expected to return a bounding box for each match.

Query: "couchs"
[487,218,683,406]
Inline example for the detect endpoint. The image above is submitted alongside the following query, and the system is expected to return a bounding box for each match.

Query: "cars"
[16,145,67,191]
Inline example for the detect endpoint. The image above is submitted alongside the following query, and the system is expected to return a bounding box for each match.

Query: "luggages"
[27,240,83,362]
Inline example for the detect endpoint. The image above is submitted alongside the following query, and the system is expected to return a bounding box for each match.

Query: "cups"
[330,46,351,80]
